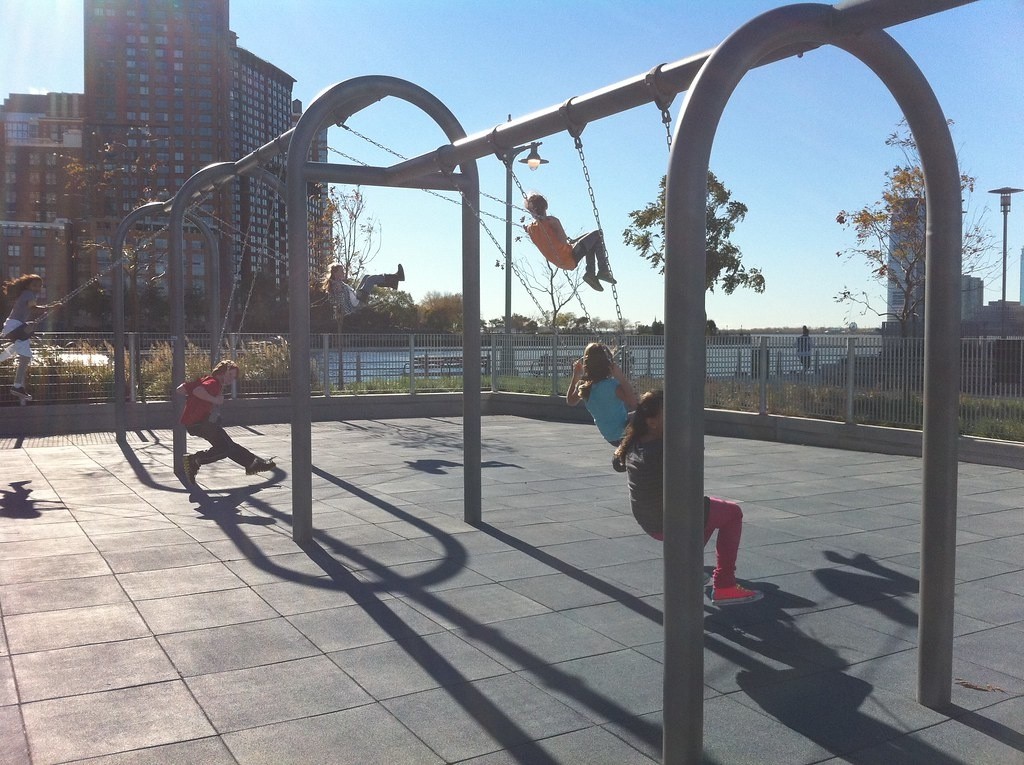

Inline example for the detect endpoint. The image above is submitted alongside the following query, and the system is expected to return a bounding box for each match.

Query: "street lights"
[495,141,550,378]
[990,186,1023,339]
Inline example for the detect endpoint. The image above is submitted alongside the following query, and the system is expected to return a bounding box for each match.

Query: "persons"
[320,262,405,319]
[175,360,277,487]
[796,326,811,374]
[612,389,764,606]
[566,342,642,446]
[523,194,617,291]
[0,273,63,402]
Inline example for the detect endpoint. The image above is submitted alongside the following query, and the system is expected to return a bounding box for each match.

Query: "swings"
[197,137,285,441]
[563,63,711,527]
[187,204,369,311]
[5,200,203,343]
[314,110,587,264]
[432,128,616,376]
